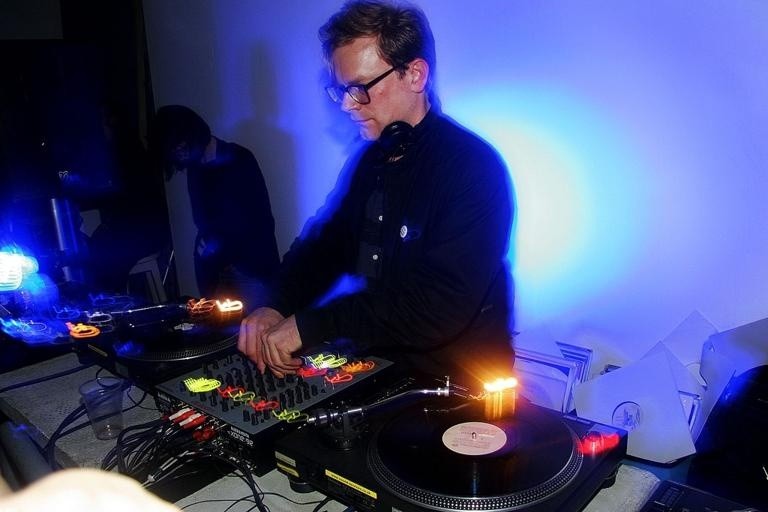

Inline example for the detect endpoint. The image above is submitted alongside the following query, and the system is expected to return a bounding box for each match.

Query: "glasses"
[323,66,396,105]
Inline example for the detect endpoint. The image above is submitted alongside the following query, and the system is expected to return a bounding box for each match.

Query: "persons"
[236,2,520,393]
[148,104,282,297]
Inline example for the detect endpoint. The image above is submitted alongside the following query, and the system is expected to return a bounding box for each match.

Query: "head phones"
[378,100,441,157]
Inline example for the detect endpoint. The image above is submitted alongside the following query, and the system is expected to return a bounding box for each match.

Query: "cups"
[79,376,125,439]
